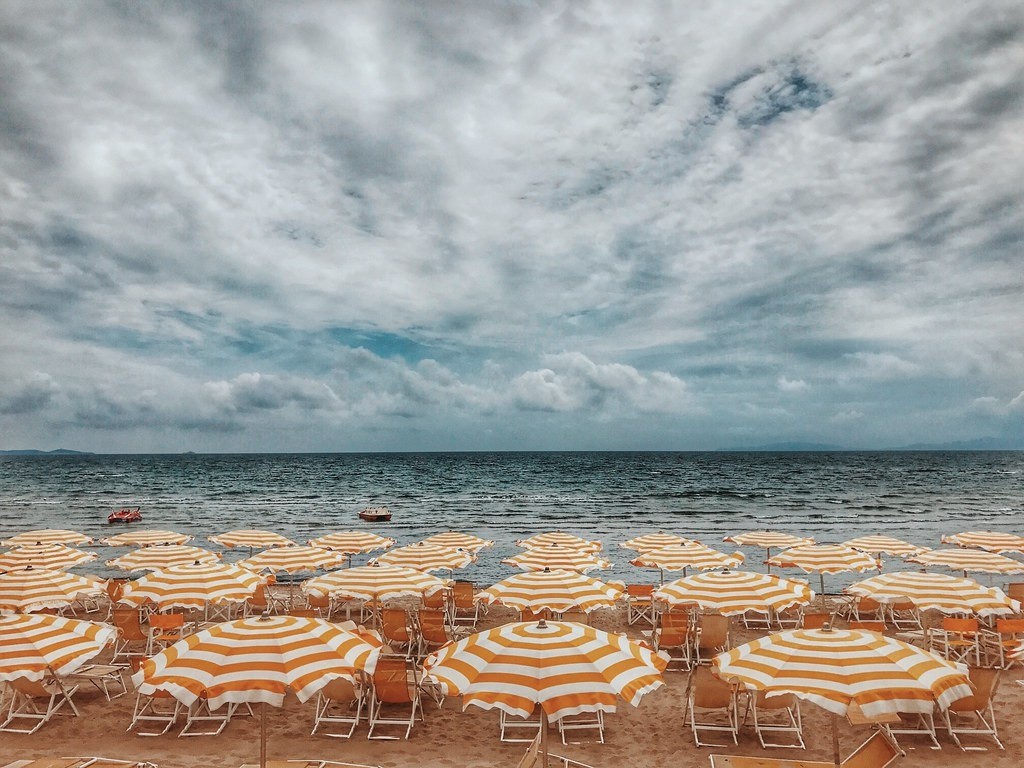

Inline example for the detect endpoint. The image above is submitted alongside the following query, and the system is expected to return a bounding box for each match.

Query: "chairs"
[0,574,1023,766]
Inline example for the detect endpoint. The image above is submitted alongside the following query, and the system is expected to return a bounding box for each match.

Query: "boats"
[108,509,143,523]
[358,507,394,521]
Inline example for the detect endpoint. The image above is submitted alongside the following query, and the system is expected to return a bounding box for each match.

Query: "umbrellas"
[0,613,117,684]
[131,610,383,768]
[709,622,979,768]
[0,527,1024,652]
[419,619,671,768]
